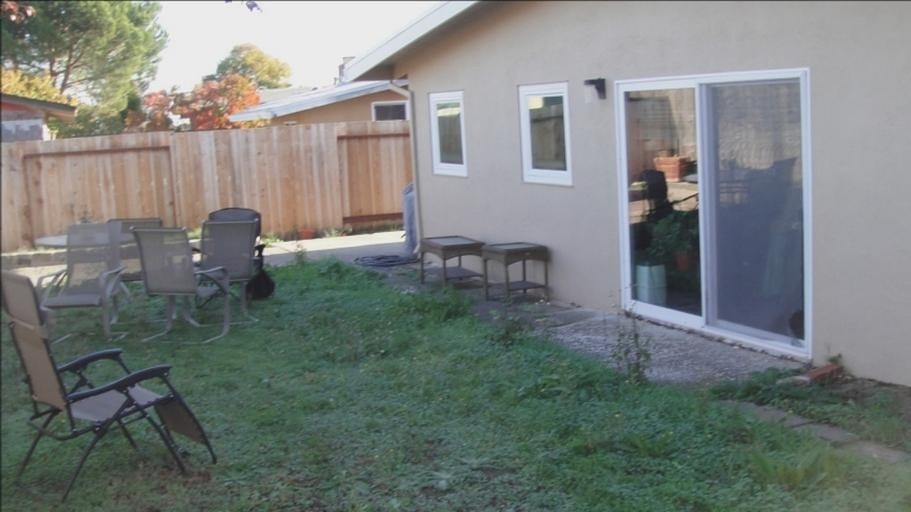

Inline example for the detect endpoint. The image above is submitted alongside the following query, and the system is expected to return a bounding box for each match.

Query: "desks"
[683,168,768,203]
[420,236,485,289]
[482,242,548,302]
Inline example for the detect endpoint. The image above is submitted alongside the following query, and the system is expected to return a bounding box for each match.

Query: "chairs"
[106,217,176,324]
[181,218,259,327]
[209,208,265,257]
[130,226,230,344]
[36,221,134,345]
[0,273,217,504]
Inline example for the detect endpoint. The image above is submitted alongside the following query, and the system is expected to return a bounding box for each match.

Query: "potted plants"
[635,212,692,307]
